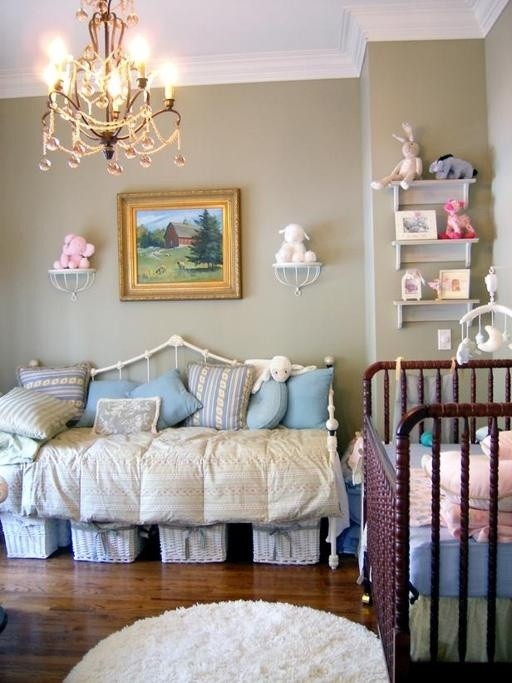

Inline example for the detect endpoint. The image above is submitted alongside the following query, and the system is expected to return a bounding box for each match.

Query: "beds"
[359,357,510,682]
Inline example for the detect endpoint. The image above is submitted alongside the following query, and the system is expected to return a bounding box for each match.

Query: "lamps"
[37,2,189,176]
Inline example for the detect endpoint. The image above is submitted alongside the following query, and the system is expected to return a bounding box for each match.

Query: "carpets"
[61,598,391,682]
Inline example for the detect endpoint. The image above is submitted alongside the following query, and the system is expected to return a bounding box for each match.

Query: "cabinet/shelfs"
[389,178,476,327]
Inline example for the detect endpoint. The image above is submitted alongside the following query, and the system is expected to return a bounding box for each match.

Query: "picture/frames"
[401,274,422,301]
[115,185,244,304]
[438,268,471,299]
[394,209,437,239]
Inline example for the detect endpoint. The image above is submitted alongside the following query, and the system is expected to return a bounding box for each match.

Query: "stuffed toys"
[275,223,317,262]
[251,355,316,394]
[371,120,422,191]
[53,234,95,270]
[429,154,478,179]
[444,198,476,235]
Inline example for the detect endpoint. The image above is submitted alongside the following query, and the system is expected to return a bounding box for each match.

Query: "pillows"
[0,359,333,442]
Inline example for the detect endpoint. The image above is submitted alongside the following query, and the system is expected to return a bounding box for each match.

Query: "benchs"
[1,334,342,568]
